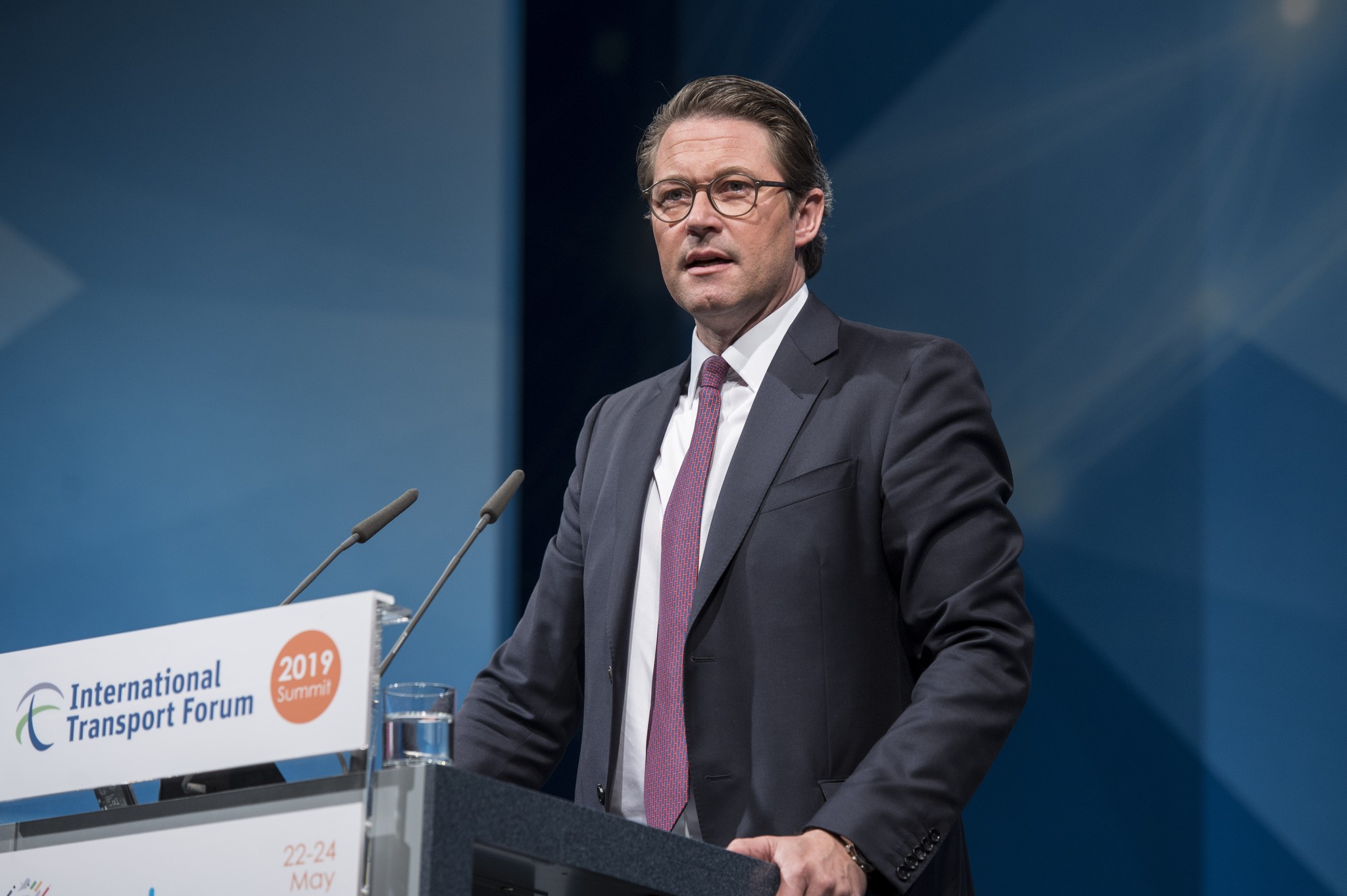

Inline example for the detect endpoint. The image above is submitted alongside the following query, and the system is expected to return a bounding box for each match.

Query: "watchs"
[840,835,876,873]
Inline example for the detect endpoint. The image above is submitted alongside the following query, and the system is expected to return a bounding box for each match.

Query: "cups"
[383,682,456,770]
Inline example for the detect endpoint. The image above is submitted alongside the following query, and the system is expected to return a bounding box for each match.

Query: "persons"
[456,76,1036,896]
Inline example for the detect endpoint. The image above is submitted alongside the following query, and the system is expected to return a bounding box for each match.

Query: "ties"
[643,356,728,833]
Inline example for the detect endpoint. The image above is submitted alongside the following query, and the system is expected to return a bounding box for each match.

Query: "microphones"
[182,488,419,793]
[351,470,525,772]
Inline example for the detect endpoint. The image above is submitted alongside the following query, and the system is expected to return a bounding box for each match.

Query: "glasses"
[641,172,803,223]
